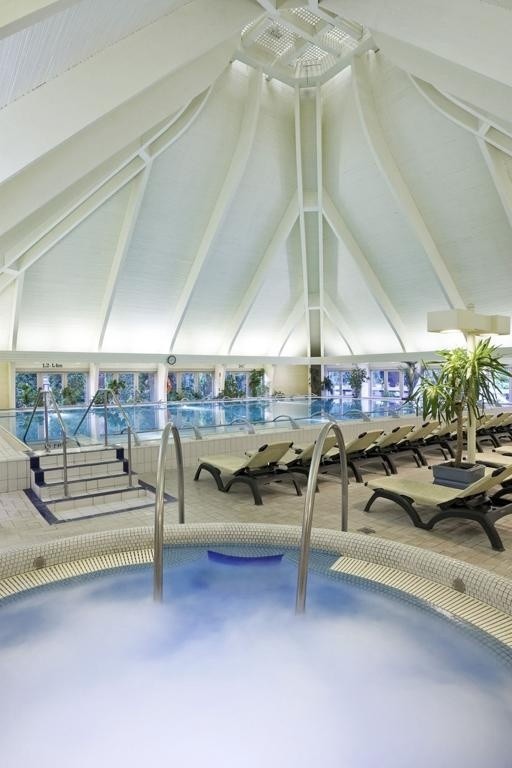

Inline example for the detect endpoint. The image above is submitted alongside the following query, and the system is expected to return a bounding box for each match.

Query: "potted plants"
[403,336,512,489]
[348,368,369,397]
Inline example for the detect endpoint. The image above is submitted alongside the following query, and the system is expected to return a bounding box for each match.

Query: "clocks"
[168,356,176,365]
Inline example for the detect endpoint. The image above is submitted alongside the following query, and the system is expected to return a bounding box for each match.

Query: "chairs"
[193,440,303,507]
[365,411,511,549]
[244,434,362,492]
[291,428,393,481]
